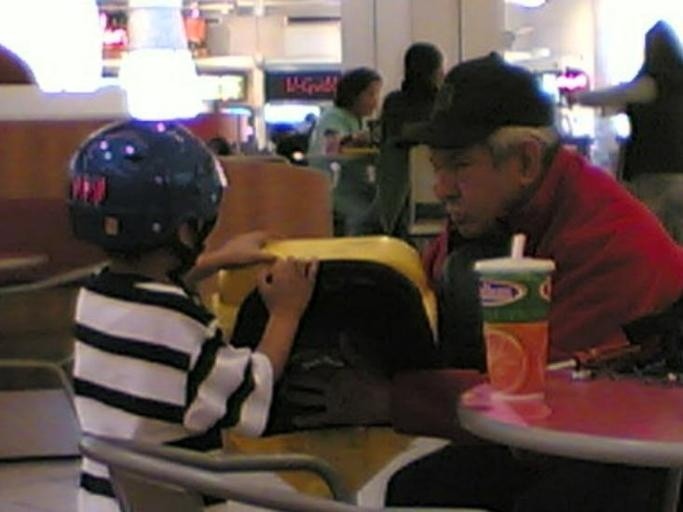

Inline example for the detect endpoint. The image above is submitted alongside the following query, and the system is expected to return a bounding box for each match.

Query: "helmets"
[67,119,228,269]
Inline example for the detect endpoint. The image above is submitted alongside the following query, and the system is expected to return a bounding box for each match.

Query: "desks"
[457,372,682,512]
[292,148,378,186]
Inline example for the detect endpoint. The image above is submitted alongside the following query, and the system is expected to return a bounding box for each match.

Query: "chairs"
[405,145,450,244]
[208,231,438,341]
[77,435,485,512]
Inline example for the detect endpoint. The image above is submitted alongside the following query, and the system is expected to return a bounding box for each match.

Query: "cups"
[472,257,554,398]
[368,121,380,148]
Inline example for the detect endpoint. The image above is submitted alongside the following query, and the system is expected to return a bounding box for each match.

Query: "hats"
[398,51,552,149]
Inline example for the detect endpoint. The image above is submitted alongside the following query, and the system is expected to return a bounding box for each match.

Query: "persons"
[289,52,682,512]
[565,21,681,245]
[368,41,445,235]
[303,67,382,232]
[66,116,322,512]
